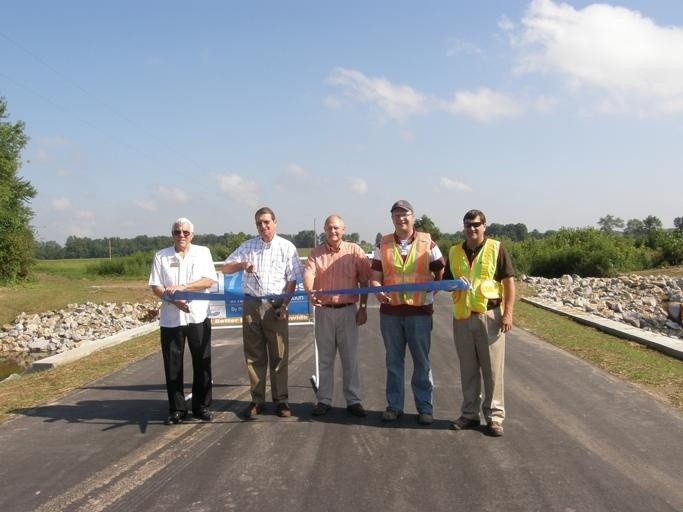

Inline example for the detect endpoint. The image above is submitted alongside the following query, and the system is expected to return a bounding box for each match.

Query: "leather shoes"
[164,408,212,424]
[245,404,504,435]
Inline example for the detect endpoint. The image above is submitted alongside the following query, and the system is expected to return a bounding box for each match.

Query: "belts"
[322,303,352,308]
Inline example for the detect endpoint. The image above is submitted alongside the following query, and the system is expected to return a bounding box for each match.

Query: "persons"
[442,209,516,436]
[372,201,446,425]
[222,207,304,420]
[303,216,373,416]
[148,217,218,425]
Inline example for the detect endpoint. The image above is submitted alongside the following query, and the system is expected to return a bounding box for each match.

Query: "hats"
[391,200,413,212]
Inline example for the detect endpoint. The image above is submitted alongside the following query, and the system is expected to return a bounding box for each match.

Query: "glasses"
[464,222,483,227]
[173,230,190,236]
[391,214,413,219]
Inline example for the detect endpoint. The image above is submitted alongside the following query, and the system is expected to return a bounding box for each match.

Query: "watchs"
[360,303,366,308]
